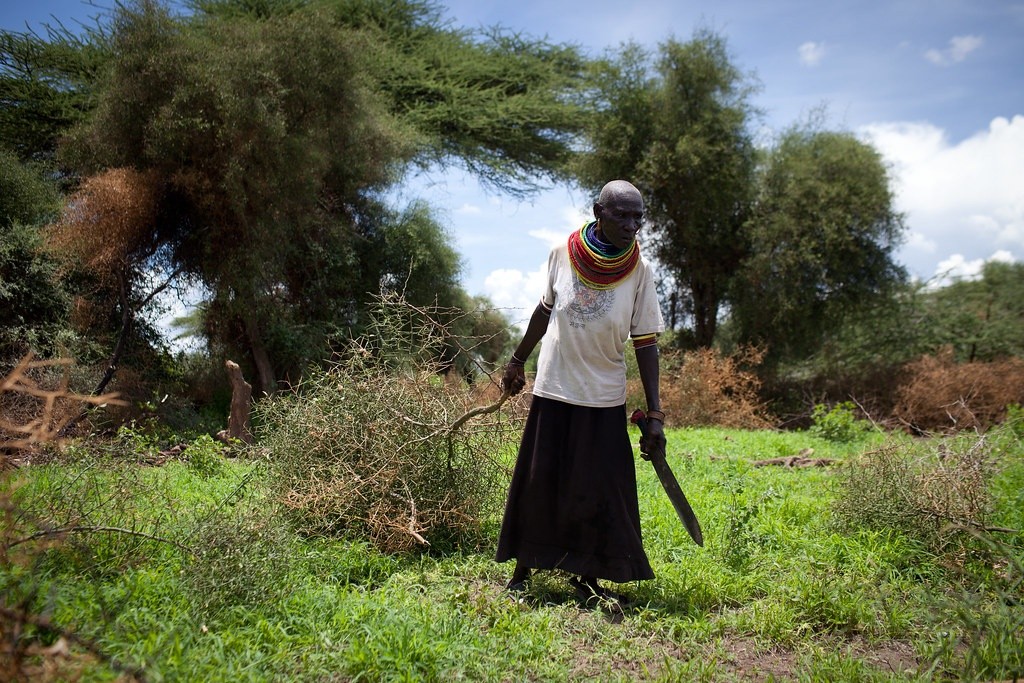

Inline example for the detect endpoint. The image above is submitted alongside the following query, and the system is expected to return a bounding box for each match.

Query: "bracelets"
[508,353,528,368]
[645,411,665,424]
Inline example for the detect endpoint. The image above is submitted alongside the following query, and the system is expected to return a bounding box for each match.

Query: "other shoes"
[570,577,628,611]
[505,568,535,598]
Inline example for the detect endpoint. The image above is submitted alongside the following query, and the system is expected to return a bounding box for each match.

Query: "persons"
[499,179,670,609]
